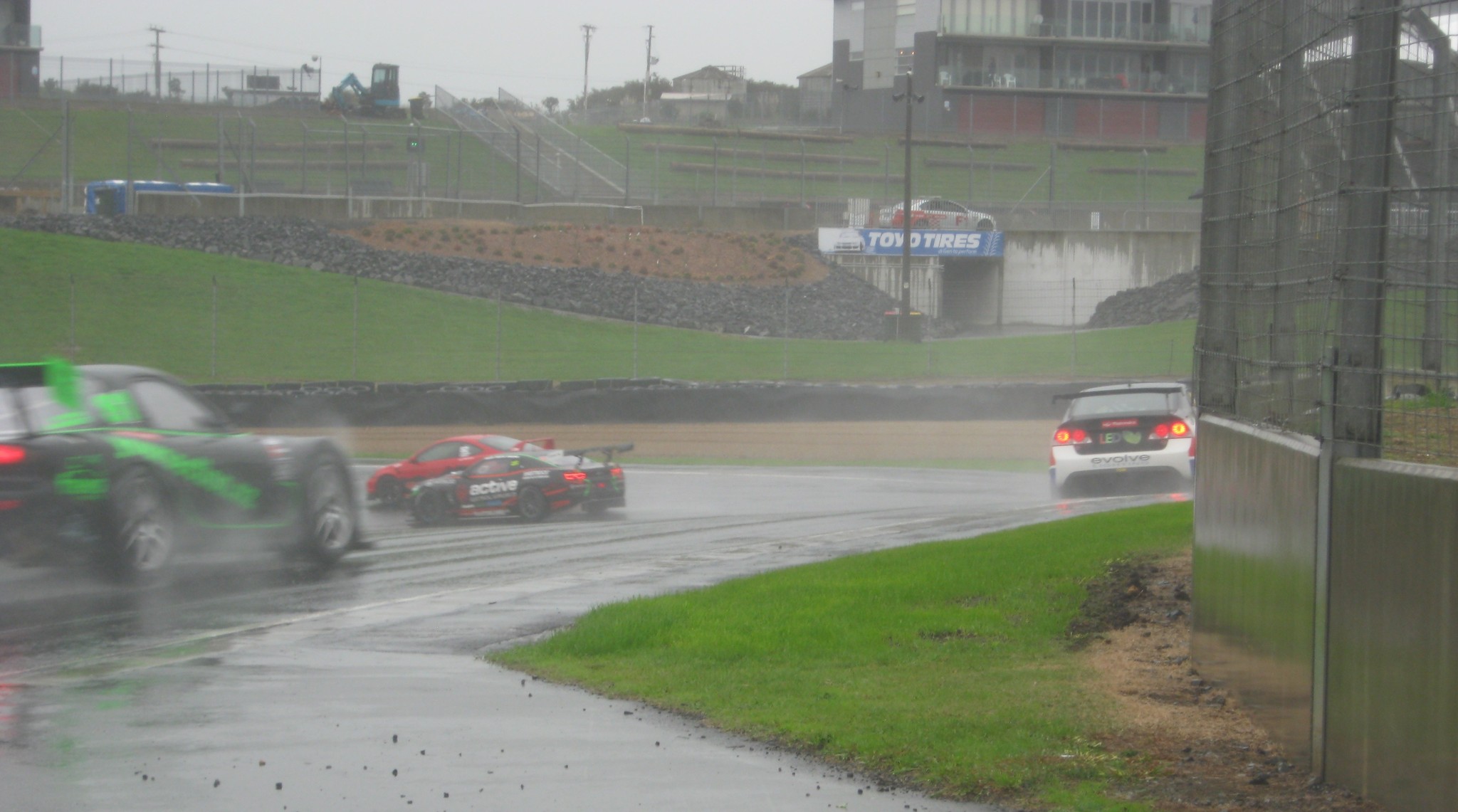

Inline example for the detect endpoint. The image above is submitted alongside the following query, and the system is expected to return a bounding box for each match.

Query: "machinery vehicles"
[330,63,407,122]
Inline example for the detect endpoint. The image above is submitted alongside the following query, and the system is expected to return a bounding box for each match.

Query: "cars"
[408,443,634,525]
[368,432,556,505]
[1048,380,1198,496]
[878,197,996,232]
[0,358,369,594]
